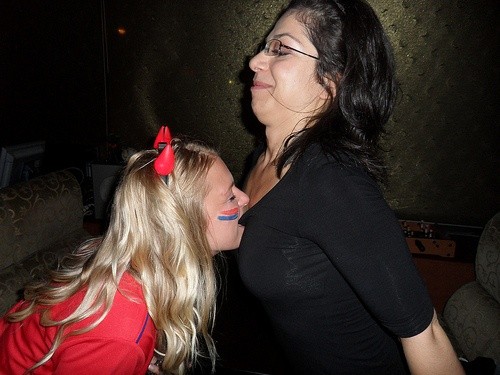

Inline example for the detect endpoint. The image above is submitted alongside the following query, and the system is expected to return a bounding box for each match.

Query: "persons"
[0,124,249,375]
[234,0,466,374]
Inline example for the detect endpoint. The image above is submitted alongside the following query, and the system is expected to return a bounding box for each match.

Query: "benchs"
[0,171,93,317]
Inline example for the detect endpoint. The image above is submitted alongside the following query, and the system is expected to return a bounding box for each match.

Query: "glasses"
[260,39,340,68]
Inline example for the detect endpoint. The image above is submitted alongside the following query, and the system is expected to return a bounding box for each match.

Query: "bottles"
[402,220,433,238]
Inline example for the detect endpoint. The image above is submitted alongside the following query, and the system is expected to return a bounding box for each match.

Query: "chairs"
[444,212,500,375]
